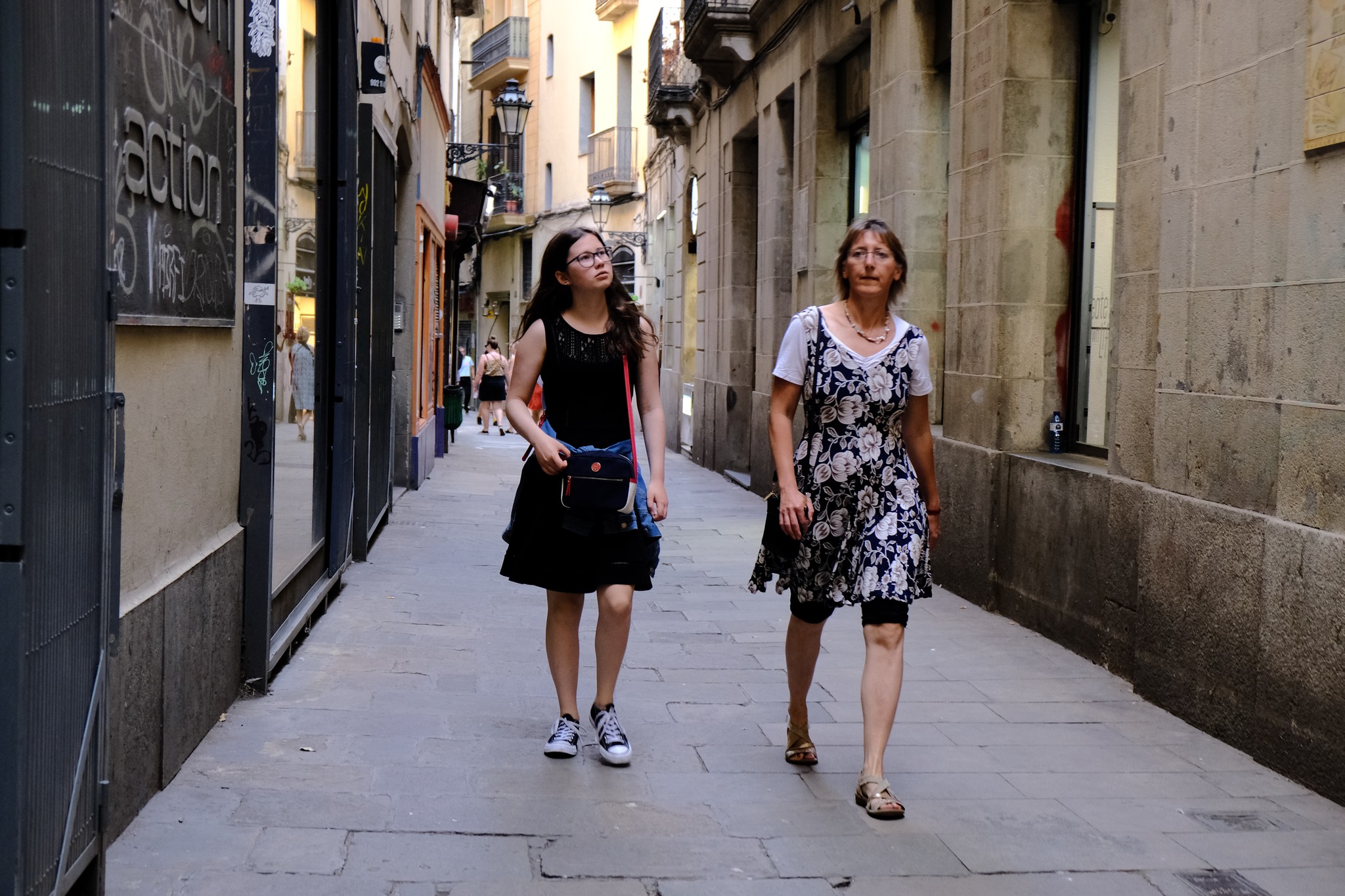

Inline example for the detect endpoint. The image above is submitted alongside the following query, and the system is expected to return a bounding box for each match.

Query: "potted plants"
[506,179,524,213]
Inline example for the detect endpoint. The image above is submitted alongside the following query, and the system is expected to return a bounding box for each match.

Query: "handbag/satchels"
[760,477,811,562]
[561,449,637,514]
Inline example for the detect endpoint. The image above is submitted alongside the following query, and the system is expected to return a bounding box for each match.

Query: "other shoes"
[499,427,505,436]
[478,430,489,435]
[493,421,498,426]
[298,423,307,441]
[477,416,482,425]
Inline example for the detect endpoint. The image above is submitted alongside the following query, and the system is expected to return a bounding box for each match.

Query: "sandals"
[785,719,818,765]
[855,770,905,817]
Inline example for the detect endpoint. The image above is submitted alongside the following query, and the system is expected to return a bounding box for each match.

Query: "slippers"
[505,430,514,433]
[515,432,519,435]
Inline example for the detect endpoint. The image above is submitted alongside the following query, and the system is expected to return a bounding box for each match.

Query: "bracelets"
[925,506,942,515]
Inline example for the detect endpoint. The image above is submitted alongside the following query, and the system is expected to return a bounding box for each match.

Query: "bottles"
[1050,411,1064,453]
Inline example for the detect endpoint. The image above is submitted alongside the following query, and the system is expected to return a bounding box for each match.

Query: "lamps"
[480,297,501,316]
[586,181,647,264]
[445,78,533,169]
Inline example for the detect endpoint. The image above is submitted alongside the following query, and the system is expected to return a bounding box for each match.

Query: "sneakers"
[544,714,588,756]
[589,702,632,765]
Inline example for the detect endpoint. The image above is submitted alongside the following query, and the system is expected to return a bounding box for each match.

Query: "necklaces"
[844,299,890,343]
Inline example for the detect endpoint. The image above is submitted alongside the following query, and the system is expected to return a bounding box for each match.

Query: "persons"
[289,328,314,440]
[449,336,544,436]
[746,220,942,818]
[497,226,668,765]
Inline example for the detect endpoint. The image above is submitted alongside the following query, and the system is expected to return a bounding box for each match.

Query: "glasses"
[566,246,613,268]
[848,249,892,263]
[485,344,489,348]
